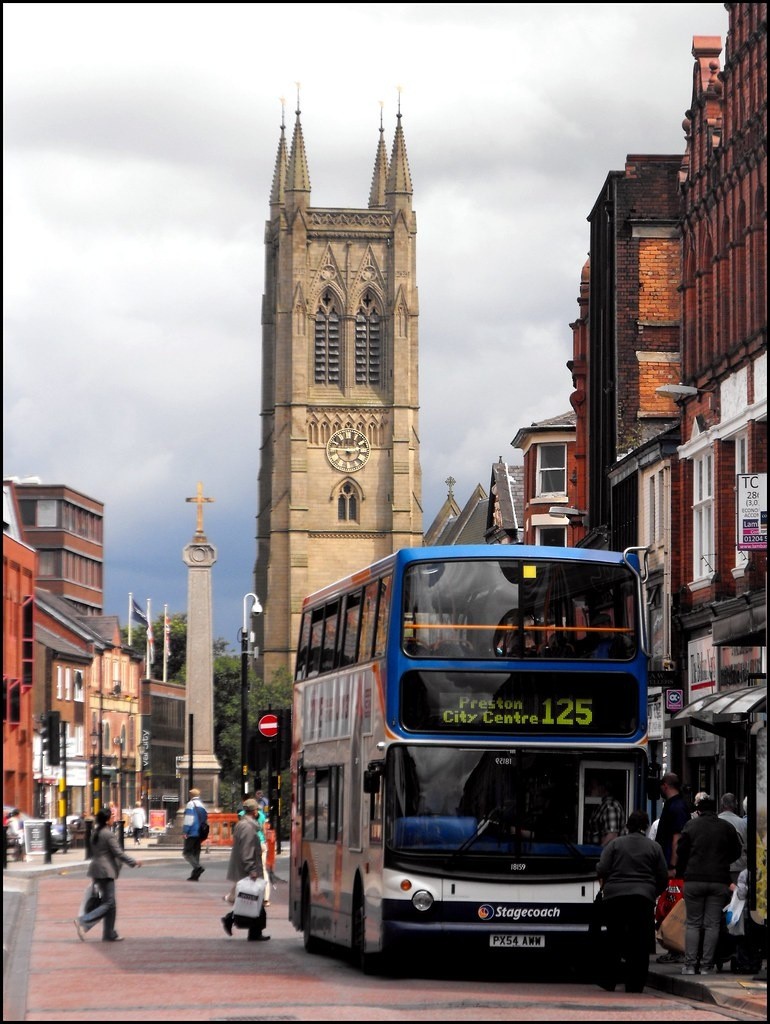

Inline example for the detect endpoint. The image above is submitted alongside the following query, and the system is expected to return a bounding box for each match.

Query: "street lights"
[90,728,97,815]
[240,592,264,800]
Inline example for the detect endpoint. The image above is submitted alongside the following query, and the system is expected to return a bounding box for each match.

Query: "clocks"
[326,428,371,474]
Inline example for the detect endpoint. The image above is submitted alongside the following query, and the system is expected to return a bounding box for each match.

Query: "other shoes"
[187,875,198,880]
[193,866,205,879]
[247,934,271,941]
[221,914,233,937]
[657,951,684,965]
[74,919,86,941]
[102,932,125,941]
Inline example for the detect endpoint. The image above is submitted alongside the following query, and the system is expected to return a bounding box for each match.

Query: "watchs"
[668,864,676,870]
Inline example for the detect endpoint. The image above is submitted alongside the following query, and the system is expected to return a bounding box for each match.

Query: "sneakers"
[681,966,695,974]
[700,969,716,975]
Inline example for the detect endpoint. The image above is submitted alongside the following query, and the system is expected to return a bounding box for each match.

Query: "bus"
[286,545,663,969]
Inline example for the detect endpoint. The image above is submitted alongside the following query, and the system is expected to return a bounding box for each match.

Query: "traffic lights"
[42,711,60,766]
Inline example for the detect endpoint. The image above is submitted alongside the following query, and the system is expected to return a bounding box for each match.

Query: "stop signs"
[257,714,277,737]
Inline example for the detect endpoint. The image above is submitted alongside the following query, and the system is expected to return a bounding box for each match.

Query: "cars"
[3,805,71,852]
[50,815,86,840]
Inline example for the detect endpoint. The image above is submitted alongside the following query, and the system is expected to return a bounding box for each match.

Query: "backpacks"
[194,805,210,841]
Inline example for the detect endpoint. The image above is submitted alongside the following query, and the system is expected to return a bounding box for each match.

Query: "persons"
[74,809,141,942]
[221,799,270,941]
[650,773,747,974]
[587,776,626,847]
[223,788,276,906]
[82,800,147,845]
[537,614,634,660]
[3,809,28,862]
[596,809,669,993]
[182,789,207,881]
[515,747,577,843]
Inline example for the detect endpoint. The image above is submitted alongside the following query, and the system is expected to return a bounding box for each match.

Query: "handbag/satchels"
[656,878,687,955]
[84,896,104,914]
[588,891,605,933]
[723,889,746,930]
[232,876,267,930]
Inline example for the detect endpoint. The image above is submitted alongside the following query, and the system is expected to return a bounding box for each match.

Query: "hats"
[188,789,200,797]
[243,799,262,811]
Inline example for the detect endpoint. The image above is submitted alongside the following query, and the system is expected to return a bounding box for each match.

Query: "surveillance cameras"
[252,603,264,616]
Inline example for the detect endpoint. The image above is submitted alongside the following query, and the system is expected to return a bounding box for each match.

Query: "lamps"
[711,573,722,584]
[549,505,587,519]
[654,384,719,400]
[743,561,758,574]
[679,585,688,595]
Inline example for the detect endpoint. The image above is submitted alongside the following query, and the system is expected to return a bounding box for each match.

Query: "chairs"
[404,636,475,658]
